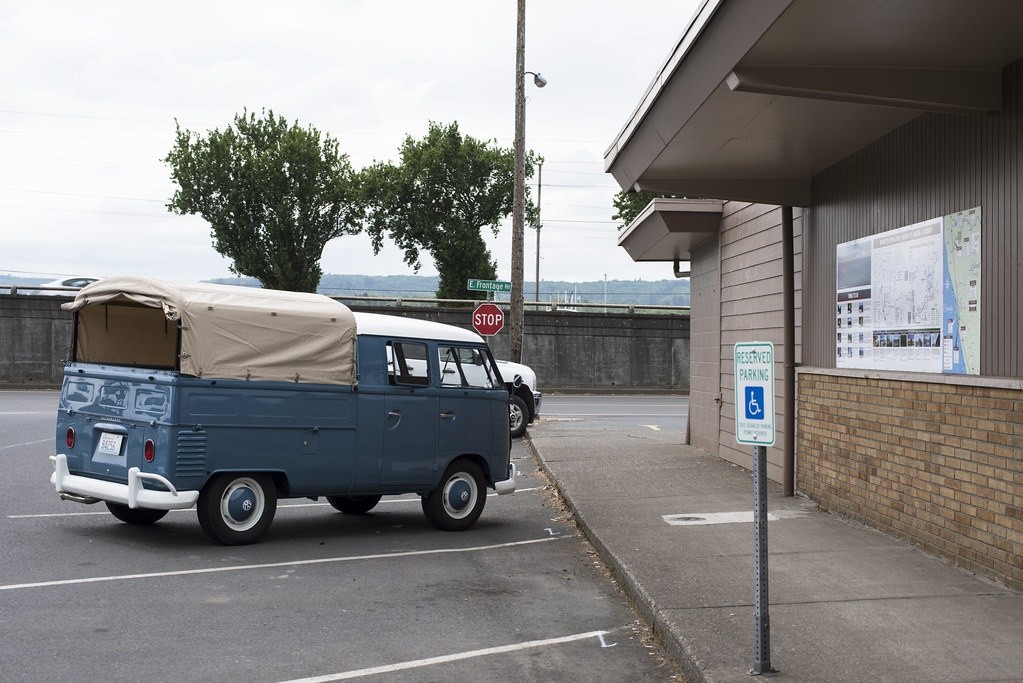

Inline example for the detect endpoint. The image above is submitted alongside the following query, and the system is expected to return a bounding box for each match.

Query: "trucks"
[47,283,523,548]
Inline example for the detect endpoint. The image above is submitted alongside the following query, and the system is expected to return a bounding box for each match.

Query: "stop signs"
[471,303,505,336]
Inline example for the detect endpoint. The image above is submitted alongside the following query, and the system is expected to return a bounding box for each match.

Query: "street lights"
[506,70,549,365]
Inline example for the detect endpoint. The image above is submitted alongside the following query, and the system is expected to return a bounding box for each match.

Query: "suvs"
[343,312,542,436]
